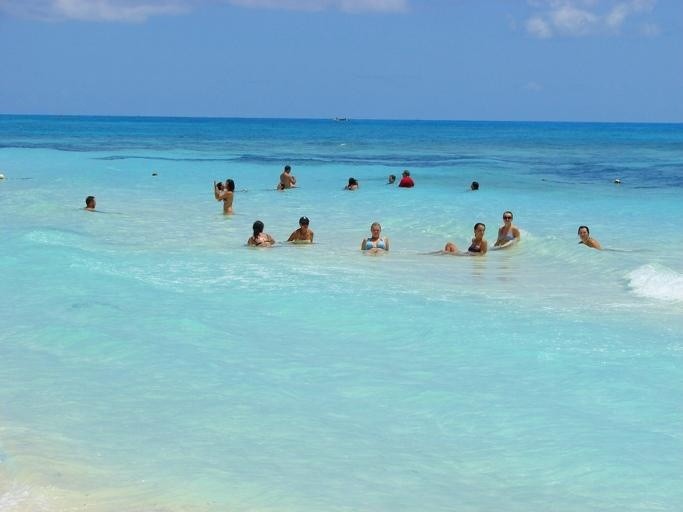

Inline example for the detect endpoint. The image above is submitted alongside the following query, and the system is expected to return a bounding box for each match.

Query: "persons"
[577,226,601,251]
[361,222,390,257]
[214,179,235,215]
[347,178,357,190]
[285,217,314,244]
[247,221,275,249]
[445,223,487,257]
[471,182,479,191]
[388,175,396,184]
[277,182,285,191]
[493,211,520,249]
[279,165,296,189]
[83,196,96,212]
[217,182,225,193]
[398,170,414,188]
